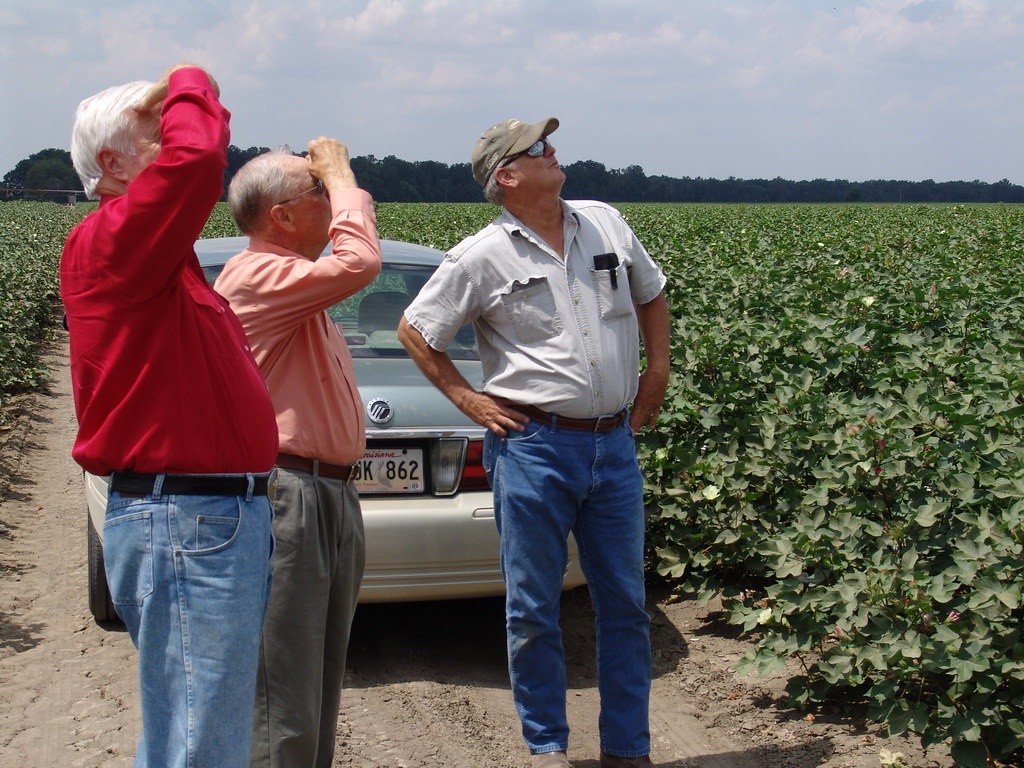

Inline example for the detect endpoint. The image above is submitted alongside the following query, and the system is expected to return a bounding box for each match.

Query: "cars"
[62,231,591,623]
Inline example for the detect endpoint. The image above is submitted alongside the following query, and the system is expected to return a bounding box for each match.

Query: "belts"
[511,405,626,434]
[111,467,280,503]
[276,452,361,485]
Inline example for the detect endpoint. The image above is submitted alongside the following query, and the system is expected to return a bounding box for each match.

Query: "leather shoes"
[599,752,655,768]
[532,749,571,768]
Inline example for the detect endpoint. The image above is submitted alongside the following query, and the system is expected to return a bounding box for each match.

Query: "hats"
[470,116,560,187]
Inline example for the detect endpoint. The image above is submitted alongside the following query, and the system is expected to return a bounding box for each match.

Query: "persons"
[60,65,276,768]
[398,119,669,768]
[212,137,383,768]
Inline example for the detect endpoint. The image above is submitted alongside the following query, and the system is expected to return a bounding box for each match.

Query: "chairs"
[357,292,415,338]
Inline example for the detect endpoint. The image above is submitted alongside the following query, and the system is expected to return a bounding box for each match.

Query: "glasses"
[279,179,323,204]
[502,136,551,167]
